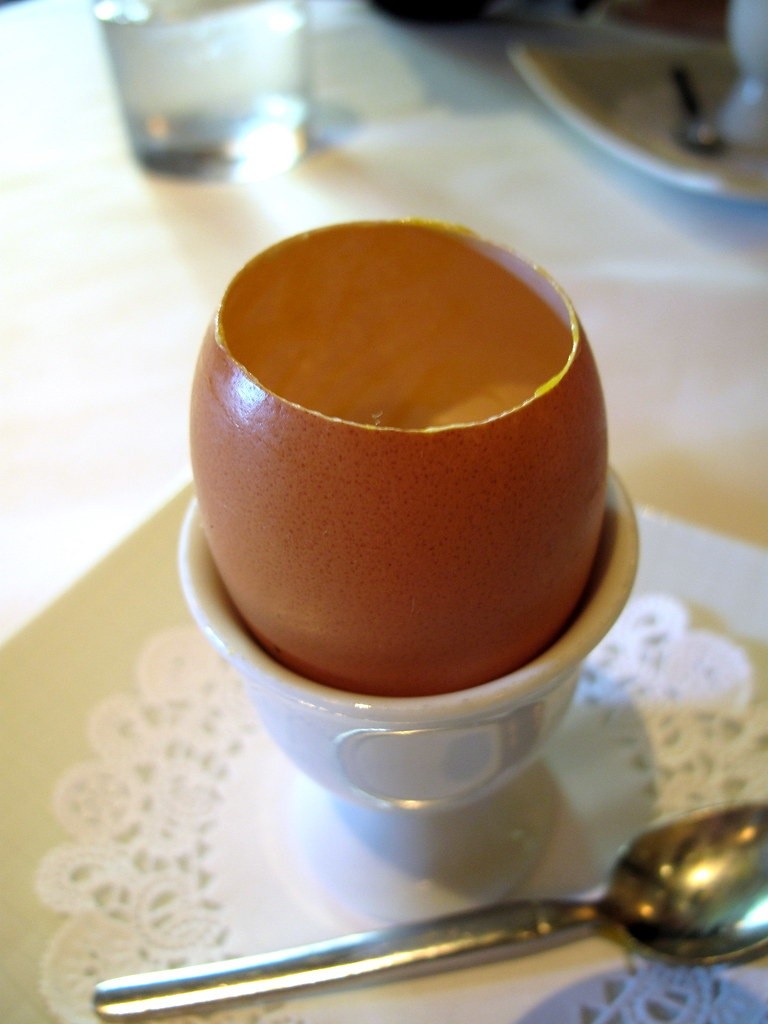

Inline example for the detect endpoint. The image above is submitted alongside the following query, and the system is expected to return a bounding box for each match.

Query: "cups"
[176,470,651,816]
[92,0,304,192]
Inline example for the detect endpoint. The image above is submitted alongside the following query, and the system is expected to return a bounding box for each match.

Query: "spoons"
[92,803,768,1019]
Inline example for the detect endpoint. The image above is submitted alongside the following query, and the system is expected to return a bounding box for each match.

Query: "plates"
[502,0,768,203]
[0,477,768,1024]
